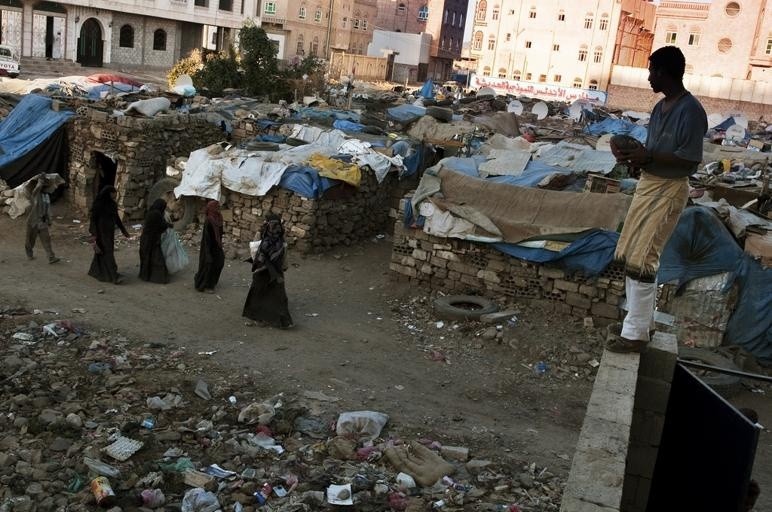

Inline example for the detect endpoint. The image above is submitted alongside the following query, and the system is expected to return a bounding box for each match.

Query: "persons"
[605,46,708,353]
[194,201,225,294]
[137,199,173,284]
[242,214,294,329]
[25,172,60,264]
[88,185,130,284]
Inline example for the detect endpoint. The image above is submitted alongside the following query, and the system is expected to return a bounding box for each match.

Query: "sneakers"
[604,322,655,353]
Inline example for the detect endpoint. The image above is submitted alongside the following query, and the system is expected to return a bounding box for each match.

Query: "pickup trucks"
[0,45,20,78]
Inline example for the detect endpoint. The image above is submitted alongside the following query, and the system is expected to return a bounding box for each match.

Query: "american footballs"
[610,134,644,166]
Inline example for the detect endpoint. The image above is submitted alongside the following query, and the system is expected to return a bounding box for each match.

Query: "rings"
[627,159,632,165]
